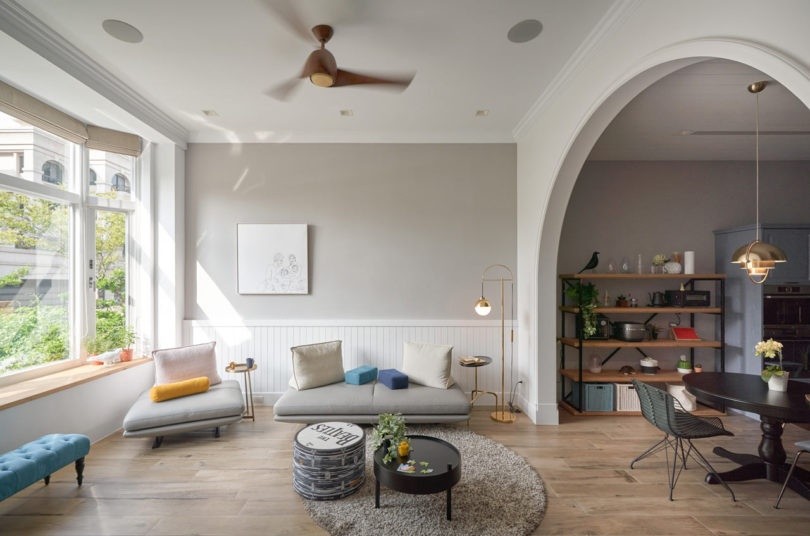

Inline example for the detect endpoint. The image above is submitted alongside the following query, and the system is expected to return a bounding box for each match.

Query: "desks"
[682,372,810,502]
[373,435,461,519]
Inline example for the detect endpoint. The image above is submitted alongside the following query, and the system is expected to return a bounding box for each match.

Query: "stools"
[458,356,498,422]
[293,421,366,497]
[123,379,246,448]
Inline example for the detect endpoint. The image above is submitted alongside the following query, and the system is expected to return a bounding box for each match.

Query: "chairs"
[630,381,737,502]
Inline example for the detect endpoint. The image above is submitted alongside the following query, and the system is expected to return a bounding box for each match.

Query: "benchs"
[1,432,91,499]
[272,380,470,425]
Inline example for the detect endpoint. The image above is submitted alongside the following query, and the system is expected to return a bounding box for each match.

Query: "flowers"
[754,338,785,383]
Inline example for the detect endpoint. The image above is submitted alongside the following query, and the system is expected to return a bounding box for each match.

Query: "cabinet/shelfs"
[560,271,725,417]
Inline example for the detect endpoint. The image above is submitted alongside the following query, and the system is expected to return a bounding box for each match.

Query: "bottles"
[634,253,644,275]
[620,257,630,273]
[589,353,601,373]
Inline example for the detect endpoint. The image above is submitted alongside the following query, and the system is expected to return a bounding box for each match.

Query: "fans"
[266,1,413,101]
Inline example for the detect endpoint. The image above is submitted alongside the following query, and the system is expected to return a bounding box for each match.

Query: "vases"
[768,370,789,391]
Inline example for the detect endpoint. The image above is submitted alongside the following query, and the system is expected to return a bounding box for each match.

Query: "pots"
[609,320,646,341]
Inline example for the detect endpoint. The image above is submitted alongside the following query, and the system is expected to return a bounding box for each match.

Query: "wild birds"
[577,251,601,274]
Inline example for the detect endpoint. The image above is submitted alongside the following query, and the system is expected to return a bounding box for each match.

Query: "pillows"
[152,340,454,401]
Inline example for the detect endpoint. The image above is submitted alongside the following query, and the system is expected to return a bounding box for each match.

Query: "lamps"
[474,265,515,423]
[731,80,787,284]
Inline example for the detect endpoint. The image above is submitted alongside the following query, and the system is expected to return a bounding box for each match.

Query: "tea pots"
[648,291,669,306]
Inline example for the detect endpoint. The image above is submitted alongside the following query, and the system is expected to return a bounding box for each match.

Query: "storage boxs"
[572,382,642,412]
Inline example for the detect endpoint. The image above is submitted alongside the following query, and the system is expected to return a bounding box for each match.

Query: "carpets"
[302,423,546,536]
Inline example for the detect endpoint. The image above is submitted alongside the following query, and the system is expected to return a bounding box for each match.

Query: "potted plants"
[565,281,599,339]
[372,410,410,464]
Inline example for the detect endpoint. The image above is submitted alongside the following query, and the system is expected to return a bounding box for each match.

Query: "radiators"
[181,320,518,406]
